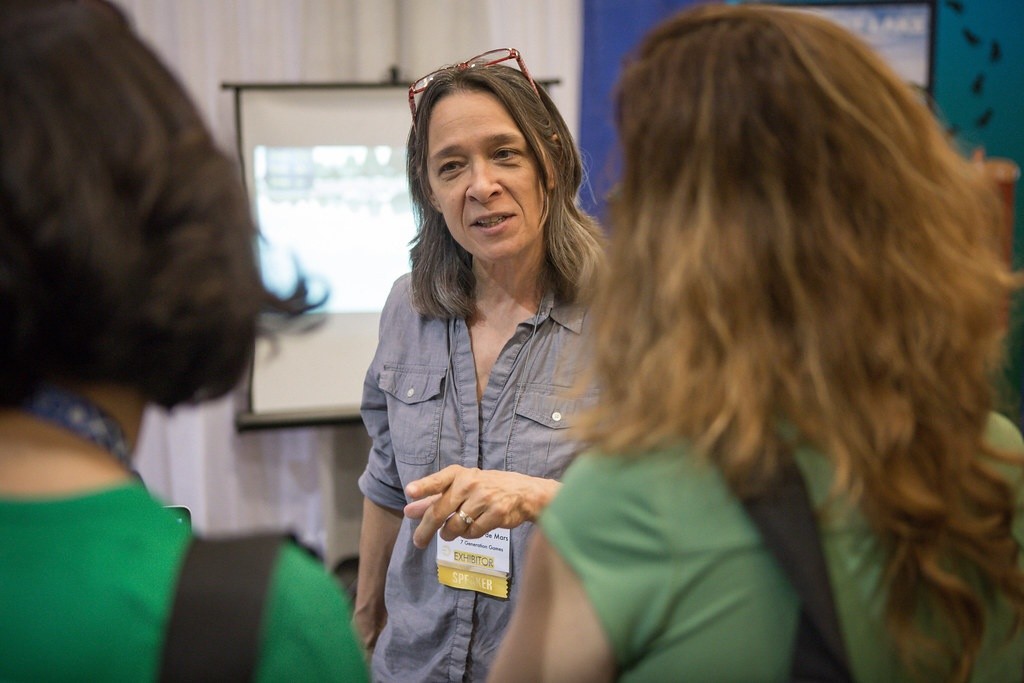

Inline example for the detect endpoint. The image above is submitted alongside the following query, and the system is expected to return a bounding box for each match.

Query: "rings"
[457,508,475,525]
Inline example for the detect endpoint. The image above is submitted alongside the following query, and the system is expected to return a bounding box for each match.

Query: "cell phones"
[163,506,193,530]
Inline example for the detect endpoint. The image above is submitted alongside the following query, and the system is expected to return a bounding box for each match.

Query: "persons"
[486,1,1021,681]
[344,65,612,678]
[3,0,377,682]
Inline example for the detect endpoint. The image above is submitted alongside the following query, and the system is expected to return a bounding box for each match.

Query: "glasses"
[407,47,541,131]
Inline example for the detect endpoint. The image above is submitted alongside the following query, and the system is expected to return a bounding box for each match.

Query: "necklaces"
[16,381,134,470]
